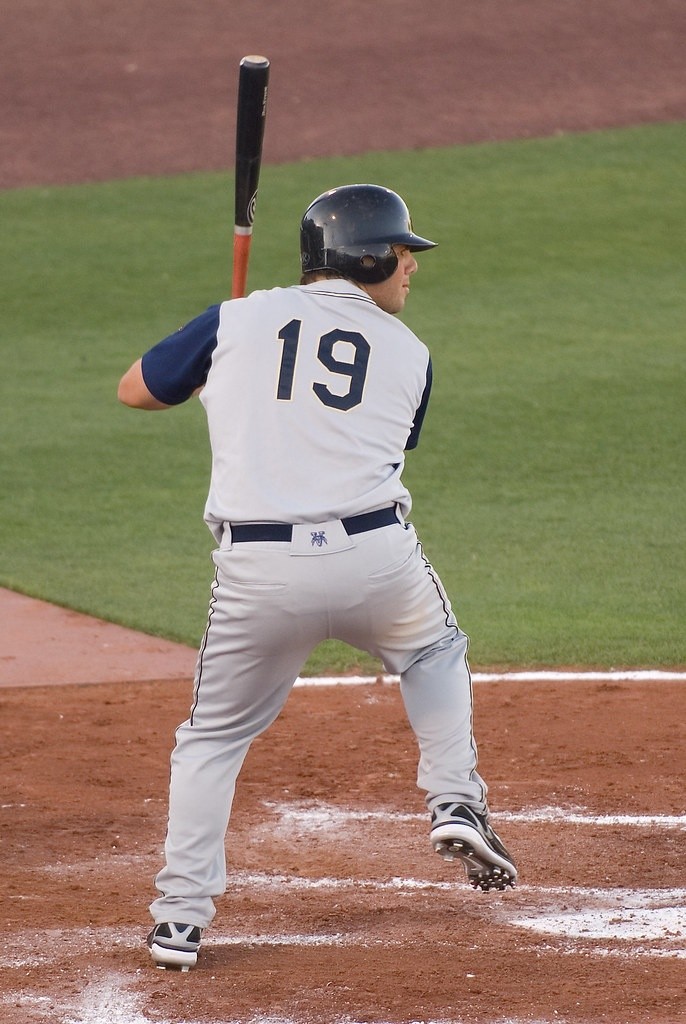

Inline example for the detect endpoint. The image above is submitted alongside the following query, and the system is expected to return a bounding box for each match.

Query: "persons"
[117,184,518,971]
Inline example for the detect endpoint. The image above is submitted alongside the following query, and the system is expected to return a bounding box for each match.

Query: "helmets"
[300,184,439,284]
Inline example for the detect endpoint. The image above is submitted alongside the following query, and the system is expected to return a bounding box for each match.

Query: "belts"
[230,505,399,544]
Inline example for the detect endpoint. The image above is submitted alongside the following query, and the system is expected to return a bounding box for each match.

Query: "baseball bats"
[231,55,270,299]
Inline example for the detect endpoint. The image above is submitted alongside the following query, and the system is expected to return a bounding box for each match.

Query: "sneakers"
[428,803,519,893]
[146,922,203,971]
